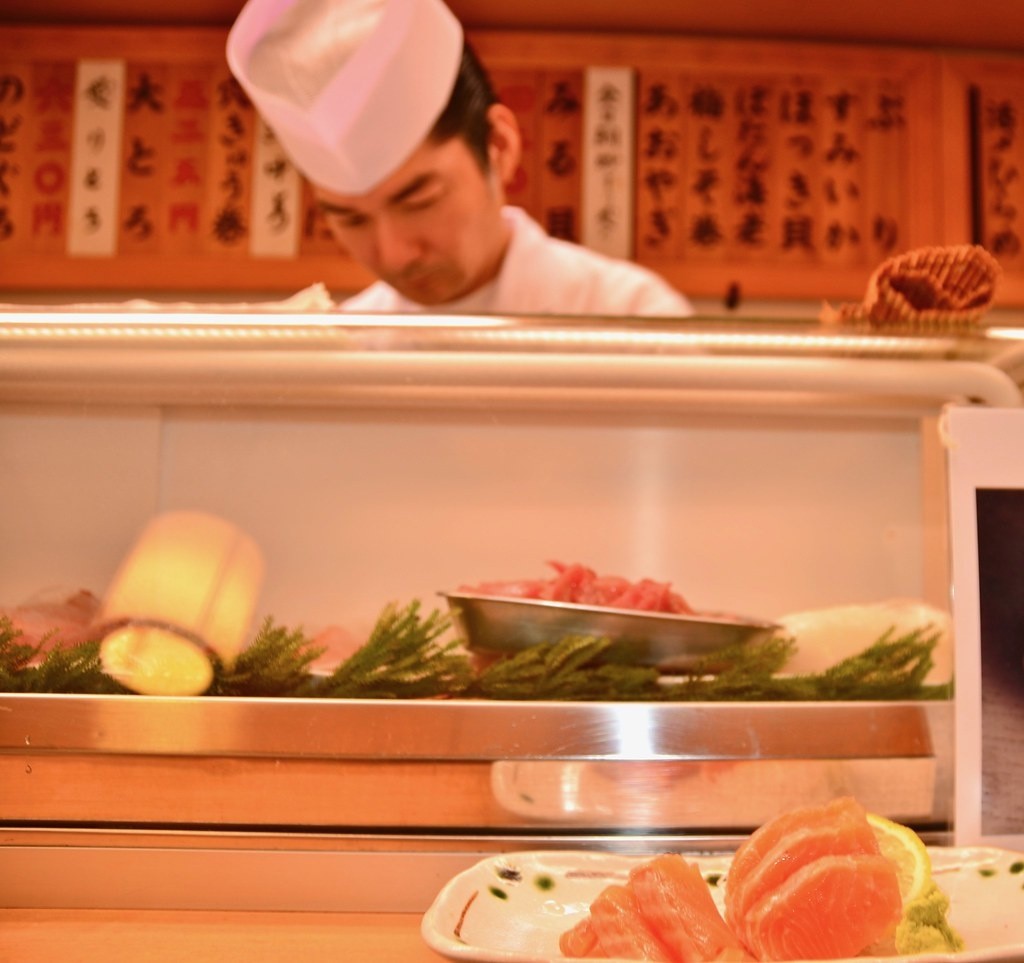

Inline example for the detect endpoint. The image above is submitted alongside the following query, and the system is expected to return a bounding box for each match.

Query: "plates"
[421,848,1024,963]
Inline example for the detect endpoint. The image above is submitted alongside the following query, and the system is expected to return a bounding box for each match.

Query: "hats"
[225,1,465,196]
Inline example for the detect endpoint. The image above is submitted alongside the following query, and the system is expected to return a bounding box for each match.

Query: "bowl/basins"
[438,589,779,670]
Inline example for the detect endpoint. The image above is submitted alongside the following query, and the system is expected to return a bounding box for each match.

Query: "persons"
[226,0,693,318]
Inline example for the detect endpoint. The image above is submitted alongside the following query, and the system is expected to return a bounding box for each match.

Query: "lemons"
[866,814,930,909]
